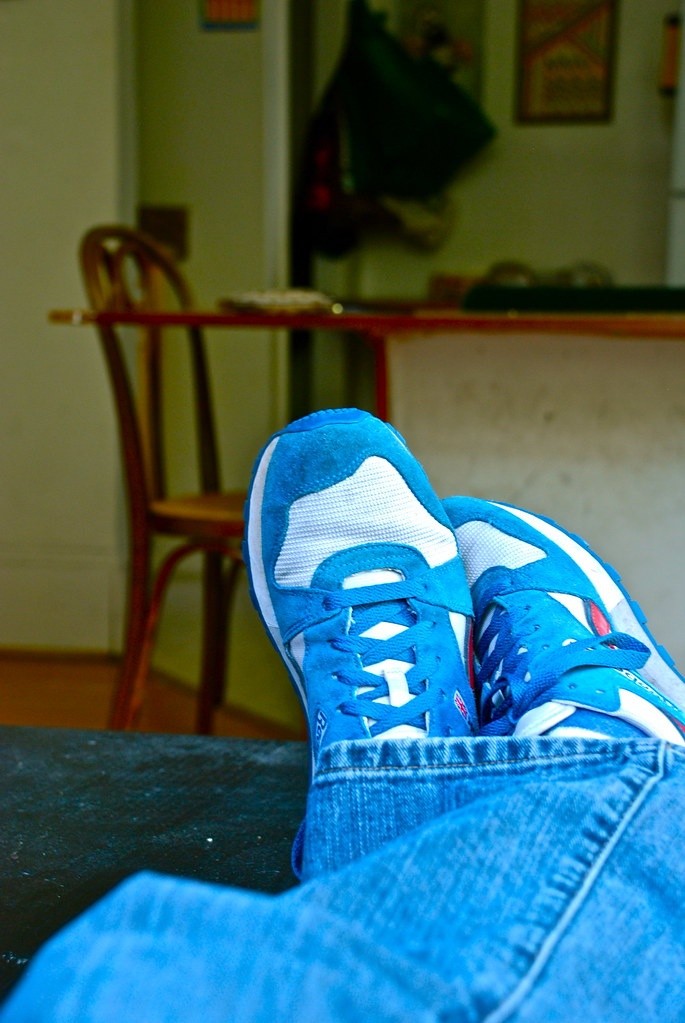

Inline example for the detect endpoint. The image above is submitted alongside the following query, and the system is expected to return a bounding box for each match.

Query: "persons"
[0,407,685,1023]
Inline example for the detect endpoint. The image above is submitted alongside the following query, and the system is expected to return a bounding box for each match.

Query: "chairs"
[80,225,250,738]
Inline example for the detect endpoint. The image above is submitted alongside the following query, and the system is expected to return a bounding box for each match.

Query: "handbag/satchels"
[292,0,499,259]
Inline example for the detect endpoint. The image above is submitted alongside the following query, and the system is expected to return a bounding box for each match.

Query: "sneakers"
[442,496,685,747]
[242,407,480,880]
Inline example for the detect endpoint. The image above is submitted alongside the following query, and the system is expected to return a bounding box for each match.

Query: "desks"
[51,307,685,678]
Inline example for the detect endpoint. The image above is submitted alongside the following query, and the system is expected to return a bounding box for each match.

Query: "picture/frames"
[512,0,621,127]
